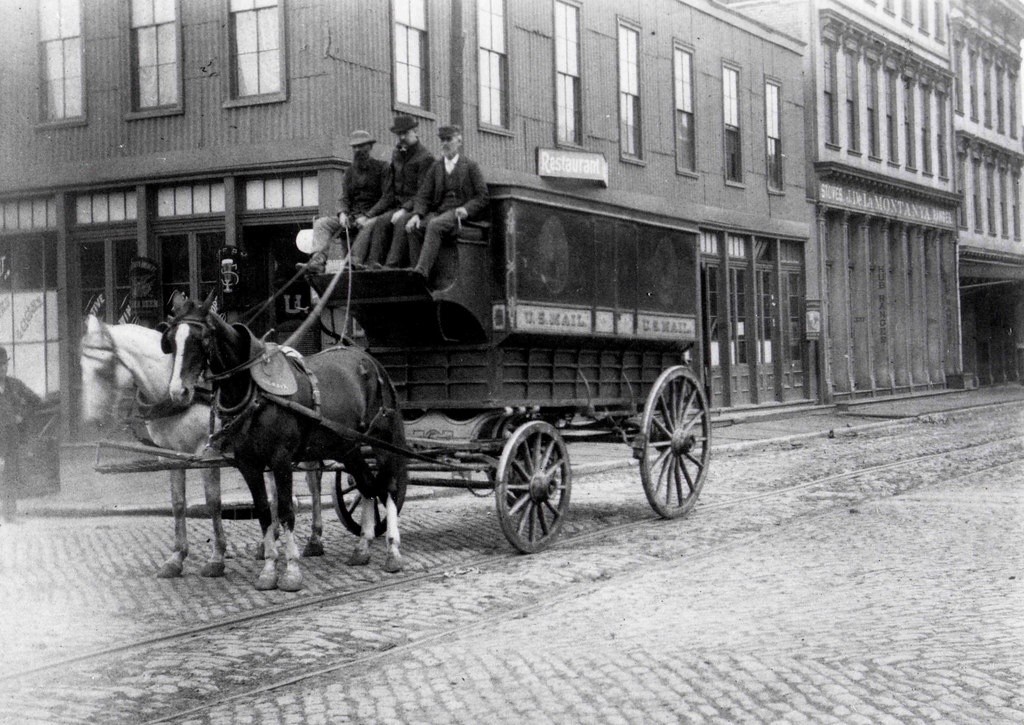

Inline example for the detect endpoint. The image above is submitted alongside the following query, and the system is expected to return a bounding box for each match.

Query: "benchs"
[326,213,486,278]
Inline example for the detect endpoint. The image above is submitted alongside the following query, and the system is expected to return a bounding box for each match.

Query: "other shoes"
[295,253,326,274]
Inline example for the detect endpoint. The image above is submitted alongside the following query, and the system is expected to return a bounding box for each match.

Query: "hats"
[390,116,419,132]
[349,131,376,147]
[438,125,460,136]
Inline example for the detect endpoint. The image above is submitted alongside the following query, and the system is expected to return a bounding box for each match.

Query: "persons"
[0,348,42,523]
[295,115,489,276]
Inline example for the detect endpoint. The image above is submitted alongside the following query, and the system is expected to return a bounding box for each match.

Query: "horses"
[79,289,405,591]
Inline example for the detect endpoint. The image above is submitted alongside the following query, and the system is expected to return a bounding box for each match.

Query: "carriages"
[76,181,713,597]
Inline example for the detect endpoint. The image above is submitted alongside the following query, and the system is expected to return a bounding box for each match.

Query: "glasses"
[440,136,458,141]
